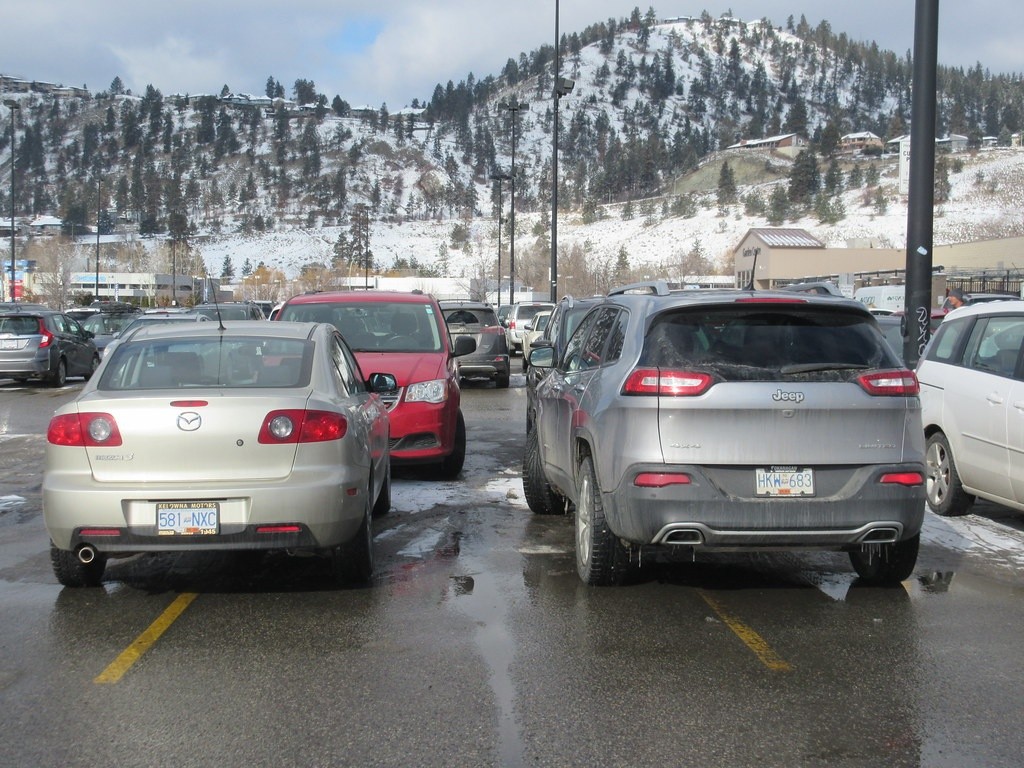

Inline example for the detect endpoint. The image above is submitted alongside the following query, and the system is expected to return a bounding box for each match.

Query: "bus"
[435,299,511,388]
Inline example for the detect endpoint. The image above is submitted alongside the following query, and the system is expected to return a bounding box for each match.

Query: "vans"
[854,285,906,313]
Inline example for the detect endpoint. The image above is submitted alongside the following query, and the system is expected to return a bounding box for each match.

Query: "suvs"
[526,293,606,435]
[503,300,556,358]
[522,280,927,589]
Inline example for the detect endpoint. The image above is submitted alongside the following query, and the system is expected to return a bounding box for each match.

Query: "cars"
[41,320,396,593]
[263,289,477,479]
[1,308,101,388]
[962,293,1021,306]
[914,298,1024,518]
[0,300,296,367]
[496,304,513,325]
[873,309,949,368]
[522,310,551,373]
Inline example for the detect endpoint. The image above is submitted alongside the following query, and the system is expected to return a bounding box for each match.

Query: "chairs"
[164,352,203,385]
[385,312,423,351]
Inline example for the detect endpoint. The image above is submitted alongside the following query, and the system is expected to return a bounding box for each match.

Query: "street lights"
[489,174,511,308]
[2,97,19,302]
[497,101,530,305]
[557,274,574,296]
[170,208,176,301]
[549,76,576,303]
[94,177,104,299]
[363,205,371,290]
